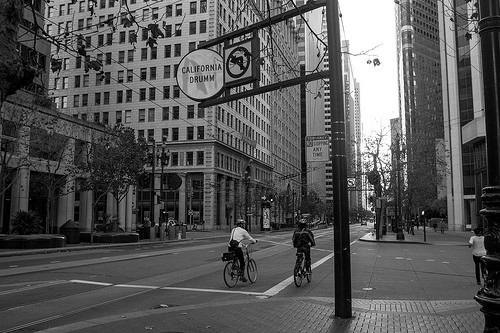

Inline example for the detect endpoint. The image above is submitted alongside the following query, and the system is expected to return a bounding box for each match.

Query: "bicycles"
[293,244,316,287]
[222,240,258,287]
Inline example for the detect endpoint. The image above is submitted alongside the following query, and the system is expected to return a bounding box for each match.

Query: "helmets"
[236,219,247,224]
[299,219,307,223]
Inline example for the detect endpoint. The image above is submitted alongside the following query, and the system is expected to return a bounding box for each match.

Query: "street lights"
[155,141,171,241]
[241,170,252,231]
[390,133,408,240]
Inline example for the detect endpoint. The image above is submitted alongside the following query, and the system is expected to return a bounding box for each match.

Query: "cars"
[361,220,367,226]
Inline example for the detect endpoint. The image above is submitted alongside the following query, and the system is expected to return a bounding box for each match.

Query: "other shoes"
[477,282,481,285]
[305,271,312,275]
[231,266,238,270]
[239,277,247,282]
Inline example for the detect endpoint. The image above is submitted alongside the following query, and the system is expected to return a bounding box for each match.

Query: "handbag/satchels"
[230,239,239,249]
[293,238,301,248]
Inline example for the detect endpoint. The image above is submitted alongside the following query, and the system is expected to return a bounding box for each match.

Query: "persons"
[440,219,446,234]
[227,217,257,284]
[468,226,490,286]
[432,220,438,232]
[291,218,315,275]
[104,214,113,231]
[161,219,184,236]
